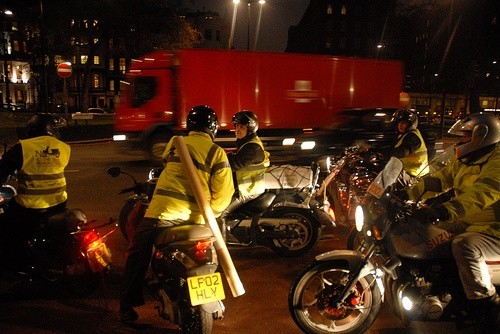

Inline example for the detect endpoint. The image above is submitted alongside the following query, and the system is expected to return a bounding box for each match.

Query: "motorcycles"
[106,154,498,333]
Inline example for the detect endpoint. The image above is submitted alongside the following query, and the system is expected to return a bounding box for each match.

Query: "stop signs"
[57,63,72,78]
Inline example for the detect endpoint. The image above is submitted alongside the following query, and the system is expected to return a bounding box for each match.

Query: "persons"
[0,113,71,275]
[216,110,270,238]
[119,105,236,327]
[364,109,430,180]
[397,111,500,317]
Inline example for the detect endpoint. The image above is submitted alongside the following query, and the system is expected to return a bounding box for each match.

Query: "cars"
[87,107,110,116]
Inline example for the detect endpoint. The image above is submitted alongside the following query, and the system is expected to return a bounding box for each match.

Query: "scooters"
[0,176,122,294]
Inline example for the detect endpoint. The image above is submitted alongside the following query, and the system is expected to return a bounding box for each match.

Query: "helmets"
[186,105,219,141]
[447,112,500,160]
[232,110,258,136]
[391,109,418,135]
[27,112,56,139]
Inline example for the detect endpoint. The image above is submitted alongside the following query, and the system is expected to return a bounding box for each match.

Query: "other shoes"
[0,276,20,293]
[469,293,500,334]
[119,308,139,324]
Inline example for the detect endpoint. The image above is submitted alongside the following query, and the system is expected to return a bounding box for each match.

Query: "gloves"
[393,190,409,201]
[363,151,382,163]
[412,204,449,225]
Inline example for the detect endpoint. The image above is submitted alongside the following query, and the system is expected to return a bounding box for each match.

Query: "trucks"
[113,52,408,146]
